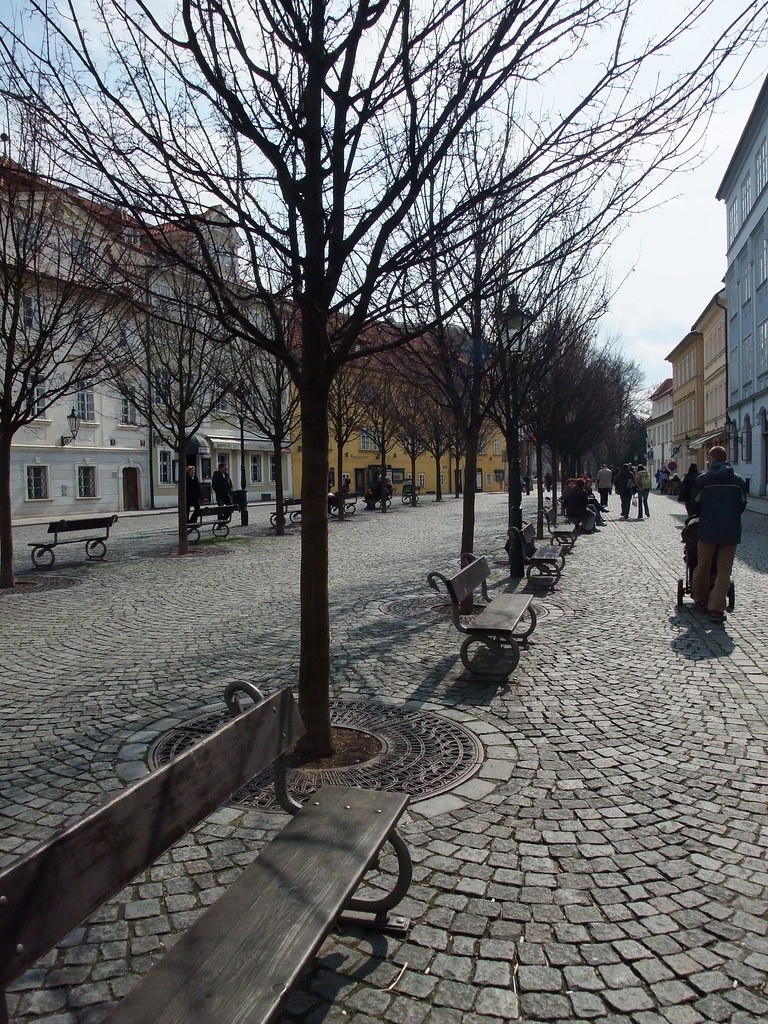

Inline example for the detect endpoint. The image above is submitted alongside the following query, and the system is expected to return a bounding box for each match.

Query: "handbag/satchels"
[632,494,638,507]
[678,487,688,502]
[627,479,638,494]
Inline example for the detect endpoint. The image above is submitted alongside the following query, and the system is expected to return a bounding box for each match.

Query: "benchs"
[328,493,360,517]
[0,679,412,1024]
[401,484,421,504]
[362,485,398,508]
[508,520,565,588]
[428,553,536,680]
[187,504,238,543]
[270,497,305,527]
[538,506,577,554]
[28,513,119,568]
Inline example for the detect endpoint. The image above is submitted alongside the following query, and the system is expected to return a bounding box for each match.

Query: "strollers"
[675,514,736,608]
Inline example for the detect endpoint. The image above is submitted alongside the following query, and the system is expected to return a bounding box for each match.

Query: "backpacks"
[640,472,651,490]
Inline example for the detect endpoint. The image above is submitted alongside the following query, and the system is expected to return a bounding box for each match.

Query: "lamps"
[723,410,738,439]
[61,406,80,446]
[685,433,693,450]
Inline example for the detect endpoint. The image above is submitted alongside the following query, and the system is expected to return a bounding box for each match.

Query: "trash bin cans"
[232,490,247,511]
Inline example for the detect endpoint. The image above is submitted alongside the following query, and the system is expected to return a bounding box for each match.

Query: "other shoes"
[593,528,600,532]
[620,512,628,519]
[711,611,727,621]
[601,508,609,512]
[596,520,607,526]
[646,512,650,517]
[696,600,709,609]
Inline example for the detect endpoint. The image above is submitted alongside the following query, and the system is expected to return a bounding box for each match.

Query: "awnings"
[210,437,291,451]
[185,434,210,454]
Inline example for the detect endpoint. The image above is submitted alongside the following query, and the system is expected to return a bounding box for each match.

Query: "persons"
[655,466,680,494]
[681,462,703,516]
[363,475,392,510]
[614,464,635,518]
[634,464,652,518]
[186,466,200,523]
[212,462,233,530]
[608,463,634,495]
[328,467,335,492]
[563,475,610,533]
[525,474,530,495]
[328,478,351,513]
[544,473,553,492]
[596,464,612,506]
[690,446,747,621]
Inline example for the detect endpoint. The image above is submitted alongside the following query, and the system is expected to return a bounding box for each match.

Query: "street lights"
[496,284,533,580]
[234,377,251,526]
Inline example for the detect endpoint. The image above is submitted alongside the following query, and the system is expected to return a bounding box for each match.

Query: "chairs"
[668,482,681,495]
